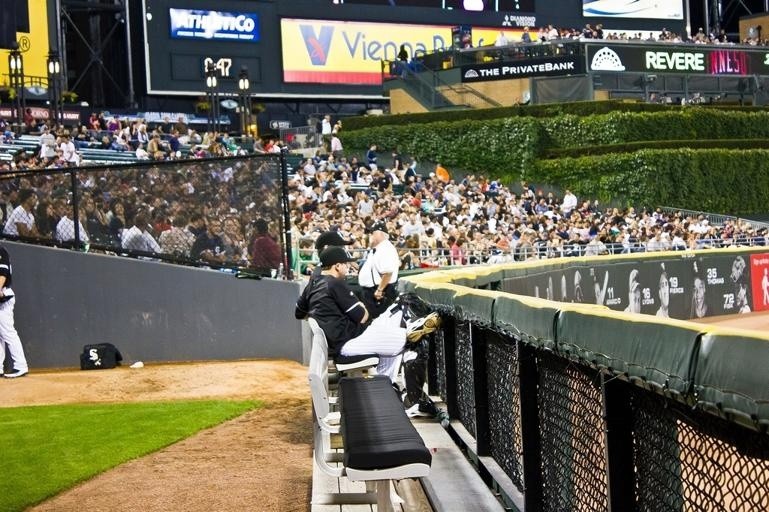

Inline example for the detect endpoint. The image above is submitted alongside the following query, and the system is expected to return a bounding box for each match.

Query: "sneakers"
[405,312,442,344]
[403,391,440,419]
[3,368,27,378]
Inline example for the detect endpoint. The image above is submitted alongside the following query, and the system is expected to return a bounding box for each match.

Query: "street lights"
[7,42,26,126]
[236,65,256,137]
[641,73,658,102]
[204,63,224,133]
[44,49,66,123]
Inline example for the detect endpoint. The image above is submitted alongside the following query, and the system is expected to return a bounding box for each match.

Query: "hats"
[251,218,268,231]
[629,269,642,293]
[315,232,356,249]
[364,219,389,234]
[319,245,359,269]
[690,258,704,280]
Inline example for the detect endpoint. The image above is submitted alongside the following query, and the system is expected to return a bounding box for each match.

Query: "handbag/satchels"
[80,342,124,370]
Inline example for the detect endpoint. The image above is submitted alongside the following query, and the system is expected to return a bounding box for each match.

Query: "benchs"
[269,152,403,194]
[1,125,254,166]
[305,330,433,512]
[306,316,379,427]
